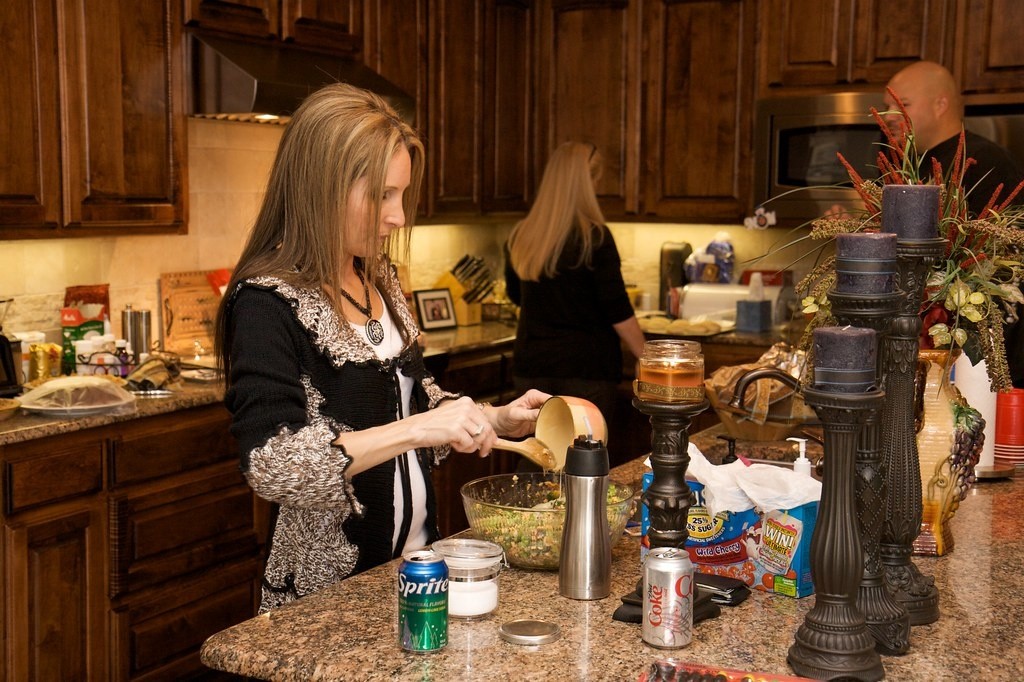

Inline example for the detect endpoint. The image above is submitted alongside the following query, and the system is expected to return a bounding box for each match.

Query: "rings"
[475,425,484,436]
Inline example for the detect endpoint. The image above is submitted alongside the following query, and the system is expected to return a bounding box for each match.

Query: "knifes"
[453,254,493,304]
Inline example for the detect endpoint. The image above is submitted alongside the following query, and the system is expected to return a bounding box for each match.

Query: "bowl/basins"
[460,473,635,571]
[0,398,22,420]
[535,396,607,471]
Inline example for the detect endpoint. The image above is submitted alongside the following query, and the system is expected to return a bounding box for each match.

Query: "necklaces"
[311,262,384,345]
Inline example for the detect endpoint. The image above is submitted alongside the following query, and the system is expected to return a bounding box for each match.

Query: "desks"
[199,415,1023,681]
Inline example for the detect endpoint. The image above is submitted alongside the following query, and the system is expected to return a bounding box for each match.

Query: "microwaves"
[753,92,1024,215]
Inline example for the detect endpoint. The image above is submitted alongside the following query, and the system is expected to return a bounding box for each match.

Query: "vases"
[915,348,987,554]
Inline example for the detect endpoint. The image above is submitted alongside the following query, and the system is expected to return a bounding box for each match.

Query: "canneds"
[640,545,694,648]
[397,549,450,655]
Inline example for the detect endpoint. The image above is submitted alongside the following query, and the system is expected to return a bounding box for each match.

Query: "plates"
[18,394,138,416]
[179,369,225,382]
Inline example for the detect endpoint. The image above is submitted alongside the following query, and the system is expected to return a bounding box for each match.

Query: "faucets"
[728,369,821,429]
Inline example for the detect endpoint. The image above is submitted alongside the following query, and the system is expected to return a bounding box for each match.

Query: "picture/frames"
[413,288,457,332]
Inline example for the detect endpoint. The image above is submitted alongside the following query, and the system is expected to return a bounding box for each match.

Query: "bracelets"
[478,402,492,410]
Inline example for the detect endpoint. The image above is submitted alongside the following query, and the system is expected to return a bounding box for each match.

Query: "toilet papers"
[951,350,998,469]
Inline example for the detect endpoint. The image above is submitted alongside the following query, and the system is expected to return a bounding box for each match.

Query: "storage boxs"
[641,468,825,600]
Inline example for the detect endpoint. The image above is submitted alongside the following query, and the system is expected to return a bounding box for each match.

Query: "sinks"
[687,442,820,477]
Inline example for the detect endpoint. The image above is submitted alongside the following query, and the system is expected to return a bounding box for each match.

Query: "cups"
[996,382,1024,469]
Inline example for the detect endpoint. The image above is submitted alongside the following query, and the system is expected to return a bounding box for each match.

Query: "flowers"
[748,81,1023,389]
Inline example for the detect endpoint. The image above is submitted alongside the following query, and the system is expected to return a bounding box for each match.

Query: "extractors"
[183,20,416,125]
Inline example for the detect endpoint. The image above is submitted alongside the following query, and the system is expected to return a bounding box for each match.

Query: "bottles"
[624,283,651,310]
[431,539,504,621]
[638,340,705,402]
[113,304,136,378]
[136,309,152,353]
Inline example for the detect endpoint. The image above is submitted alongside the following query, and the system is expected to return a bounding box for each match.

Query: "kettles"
[0,325,23,396]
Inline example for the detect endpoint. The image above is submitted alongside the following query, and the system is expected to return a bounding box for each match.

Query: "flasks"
[559,434,610,600]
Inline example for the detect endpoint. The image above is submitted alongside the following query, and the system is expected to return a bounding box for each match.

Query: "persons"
[504,139,649,482]
[213,81,552,616]
[882,60,1024,388]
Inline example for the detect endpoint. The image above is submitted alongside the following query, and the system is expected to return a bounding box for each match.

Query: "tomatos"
[699,562,796,591]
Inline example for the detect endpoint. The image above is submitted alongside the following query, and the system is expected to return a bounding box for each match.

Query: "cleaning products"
[718,434,742,463]
[784,437,814,479]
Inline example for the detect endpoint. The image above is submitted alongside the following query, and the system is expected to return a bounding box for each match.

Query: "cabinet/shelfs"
[0,331,519,682]
[0,1,1024,243]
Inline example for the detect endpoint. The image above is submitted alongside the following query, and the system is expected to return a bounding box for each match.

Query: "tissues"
[735,270,772,337]
